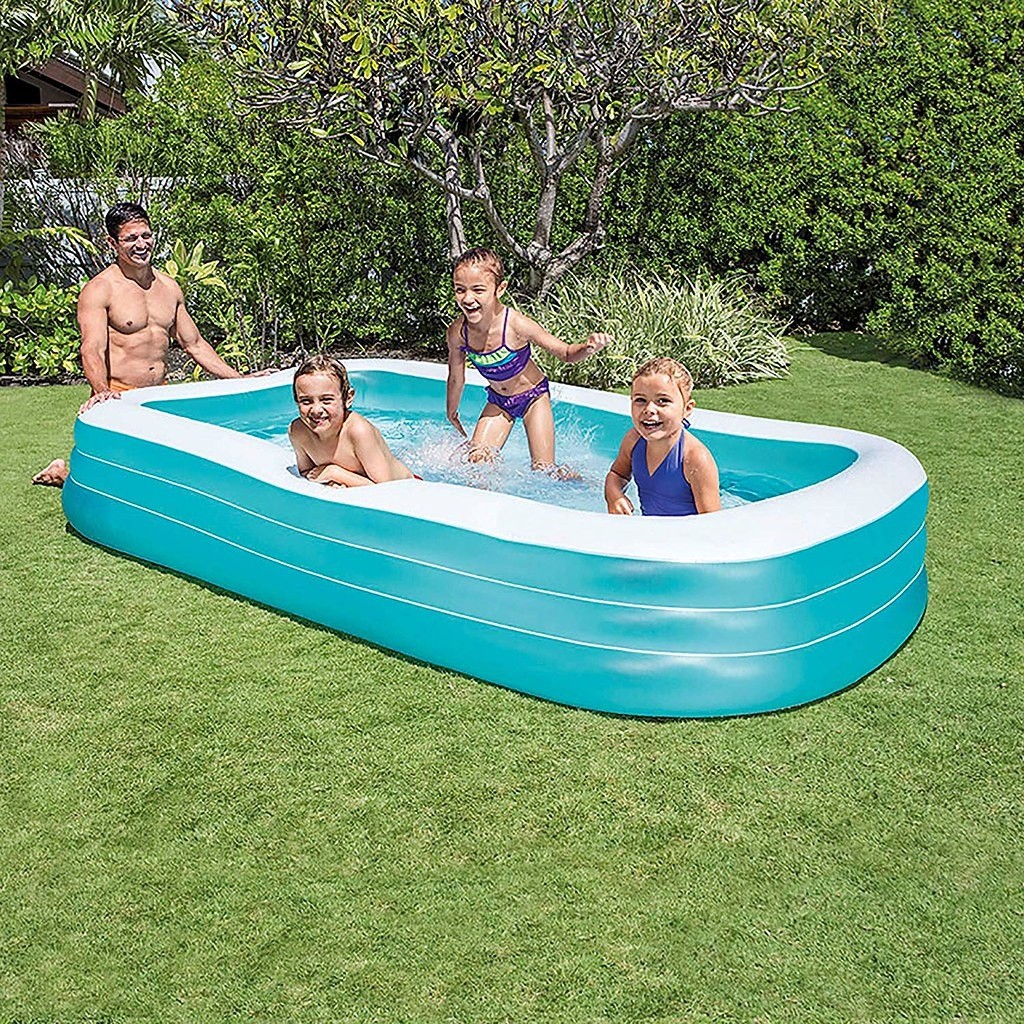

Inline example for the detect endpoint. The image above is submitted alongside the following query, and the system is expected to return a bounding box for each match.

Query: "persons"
[31,202,279,488]
[605,358,721,516]
[446,248,614,471]
[288,354,423,489]
